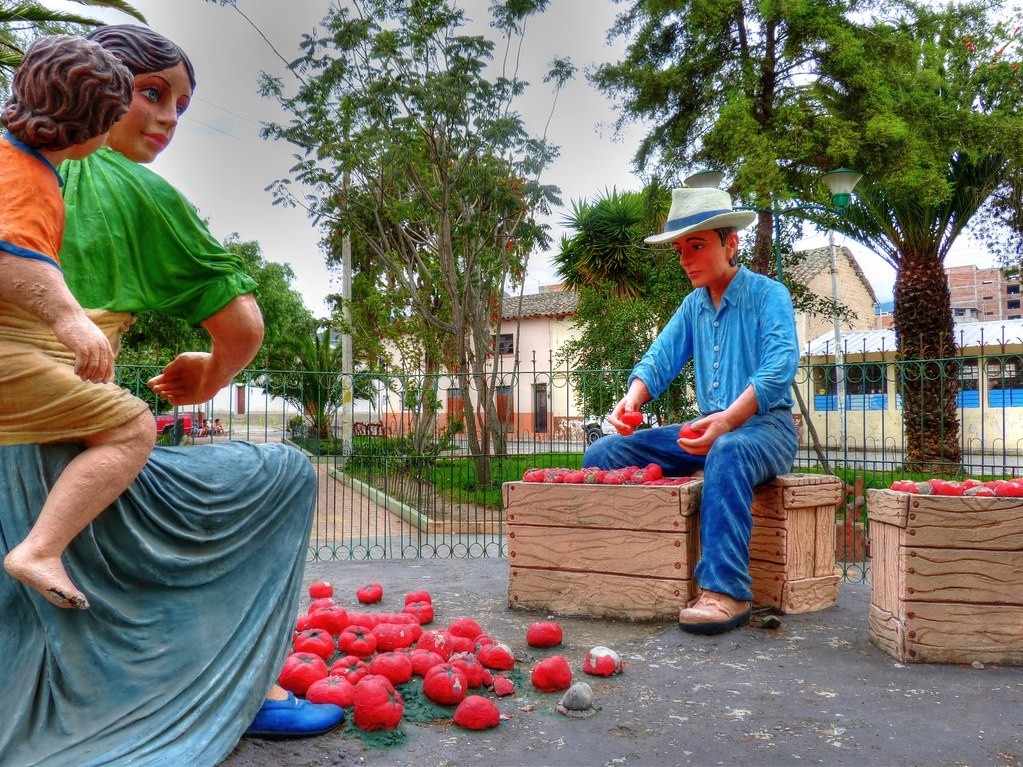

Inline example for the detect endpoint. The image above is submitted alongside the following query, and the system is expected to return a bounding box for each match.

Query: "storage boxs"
[502,477,703,621]
[867,486,1023,665]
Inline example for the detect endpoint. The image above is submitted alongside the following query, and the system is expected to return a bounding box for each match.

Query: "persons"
[582,186,798,634]
[188,417,226,439]
[53,22,265,407]
[0,33,156,611]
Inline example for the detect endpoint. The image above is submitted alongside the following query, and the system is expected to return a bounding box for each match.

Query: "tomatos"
[621,411,642,427]
[523,463,692,485]
[280,581,624,731]
[890,477,1023,497]
[679,422,706,439]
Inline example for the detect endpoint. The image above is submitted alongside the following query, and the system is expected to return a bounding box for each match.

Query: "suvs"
[580,391,666,446]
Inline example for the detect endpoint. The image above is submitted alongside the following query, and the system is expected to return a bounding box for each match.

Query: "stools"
[694,468,840,614]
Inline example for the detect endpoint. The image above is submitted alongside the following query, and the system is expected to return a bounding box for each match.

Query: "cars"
[153,409,193,437]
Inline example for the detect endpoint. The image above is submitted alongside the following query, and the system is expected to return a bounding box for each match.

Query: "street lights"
[732,164,866,285]
